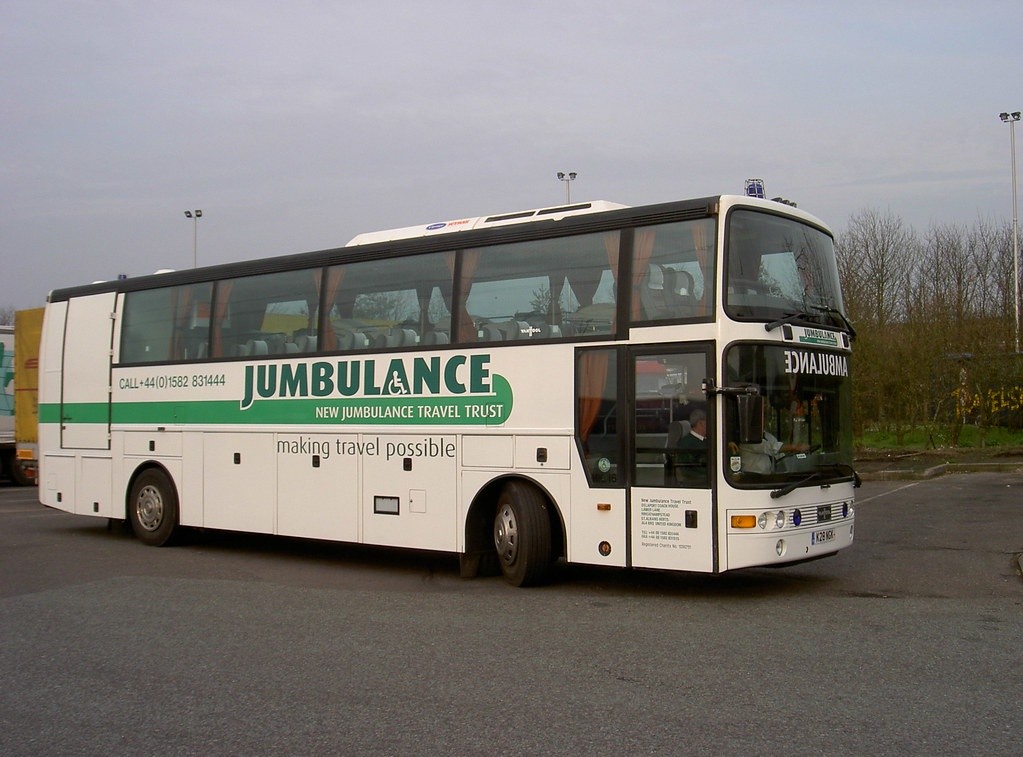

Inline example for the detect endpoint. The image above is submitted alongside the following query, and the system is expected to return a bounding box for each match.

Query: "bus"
[36,192,864,589]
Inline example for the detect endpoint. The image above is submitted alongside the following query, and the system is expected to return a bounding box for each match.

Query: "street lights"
[184,209,204,267]
[999,111,1021,353]
[557,172,577,204]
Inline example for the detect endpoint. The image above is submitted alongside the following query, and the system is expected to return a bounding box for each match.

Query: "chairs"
[172,254,803,359]
[587,418,760,487]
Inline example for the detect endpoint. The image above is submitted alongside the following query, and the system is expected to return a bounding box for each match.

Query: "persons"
[729,382,810,476]
[677,408,739,483]
